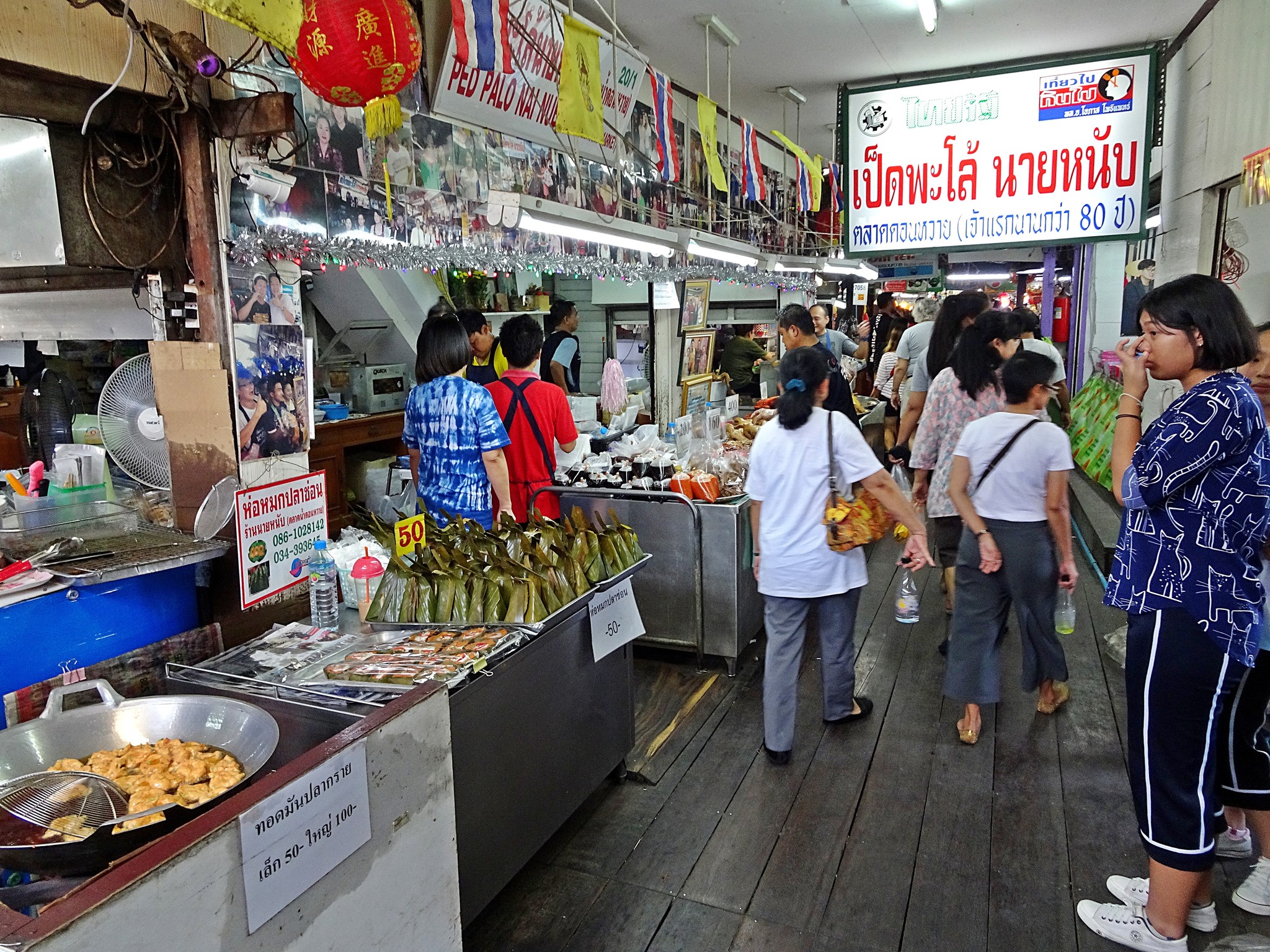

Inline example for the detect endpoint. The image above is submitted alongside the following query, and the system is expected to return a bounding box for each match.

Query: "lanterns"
[284,0,422,223]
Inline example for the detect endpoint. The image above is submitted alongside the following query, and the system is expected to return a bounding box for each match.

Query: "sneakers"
[1076,899,1191,951]
[1216,829,1253,858]
[1107,875,1219,932]
[1233,856,1270,915]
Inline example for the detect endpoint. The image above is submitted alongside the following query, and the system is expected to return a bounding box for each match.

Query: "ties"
[418,228,420,247]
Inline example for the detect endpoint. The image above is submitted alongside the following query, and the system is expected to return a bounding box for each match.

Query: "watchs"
[859,335,869,341]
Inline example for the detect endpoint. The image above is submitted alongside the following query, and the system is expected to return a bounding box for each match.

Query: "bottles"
[1054,575,1076,634]
[1100,350,1122,383]
[703,402,712,415]
[589,427,608,439]
[895,556,920,623]
[664,423,677,444]
[307,540,341,632]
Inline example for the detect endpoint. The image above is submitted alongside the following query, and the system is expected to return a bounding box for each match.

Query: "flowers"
[456,269,488,294]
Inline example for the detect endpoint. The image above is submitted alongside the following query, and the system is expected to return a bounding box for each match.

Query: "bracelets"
[282,306,285,310]
[1115,414,1142,423]
[753,553,759,556]
[1119,393,1144,412]
[977,528,990,538]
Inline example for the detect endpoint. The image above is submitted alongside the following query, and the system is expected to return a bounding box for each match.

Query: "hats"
[253,272,267,286]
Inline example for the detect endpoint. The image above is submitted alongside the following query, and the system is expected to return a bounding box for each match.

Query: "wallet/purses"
[888,445,915,473]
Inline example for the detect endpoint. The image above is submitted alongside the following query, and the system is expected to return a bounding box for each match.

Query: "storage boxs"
[12,482,110,537]
[710,379,733,401]
[582,424,638,455]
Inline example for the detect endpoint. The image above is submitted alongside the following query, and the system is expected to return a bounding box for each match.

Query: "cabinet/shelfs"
[0,387,29,471]
[308,408,416,538]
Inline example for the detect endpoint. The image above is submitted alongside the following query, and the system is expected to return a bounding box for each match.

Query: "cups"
[351,557,386,623]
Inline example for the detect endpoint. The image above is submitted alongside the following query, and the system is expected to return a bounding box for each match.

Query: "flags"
[555,11,605,145]
[697,92,728,191]
[646,63,680,182]
[770,131,842,223]
[450,0,516,73]
[740,118,765,201]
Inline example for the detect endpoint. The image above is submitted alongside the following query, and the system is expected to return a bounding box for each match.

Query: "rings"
[916,559,920,560]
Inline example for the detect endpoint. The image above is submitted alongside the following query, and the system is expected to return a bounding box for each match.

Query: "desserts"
[323,626,510,684]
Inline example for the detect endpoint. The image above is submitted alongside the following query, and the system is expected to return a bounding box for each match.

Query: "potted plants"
[526,283,544,302]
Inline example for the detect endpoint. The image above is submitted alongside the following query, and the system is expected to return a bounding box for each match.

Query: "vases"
[463,292,486,311]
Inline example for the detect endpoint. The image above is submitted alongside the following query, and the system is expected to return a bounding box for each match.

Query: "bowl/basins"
[856,395,880,407]
[314,410,326,422]
[318,404,351,420]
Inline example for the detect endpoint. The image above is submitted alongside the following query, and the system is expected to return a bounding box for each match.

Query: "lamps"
[917,0,942,36]
[756,251,825,273]
[826,258,882,280]
[667,225,764,268]
[485,191,678,258]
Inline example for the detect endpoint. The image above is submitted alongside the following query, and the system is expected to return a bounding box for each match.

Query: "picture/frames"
[676,330,716,386]
[677,277,711,338]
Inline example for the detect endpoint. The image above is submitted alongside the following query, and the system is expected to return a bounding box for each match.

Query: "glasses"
[1012,335,1022,347]
[1145,267,1155,273]
[422,312,459,328]
[238,381,253,390]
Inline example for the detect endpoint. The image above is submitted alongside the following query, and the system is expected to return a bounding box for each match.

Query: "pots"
[0,678,279,874]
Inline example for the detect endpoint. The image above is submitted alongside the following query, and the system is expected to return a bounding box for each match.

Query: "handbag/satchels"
[891,462,913,503]
[823,487,895,553]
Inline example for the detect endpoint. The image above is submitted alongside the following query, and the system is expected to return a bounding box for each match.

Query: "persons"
[750,290,1067,655]
[744,347,934,767]
[236,373,306,460]
[308,101,814,256]
[1119,259,1155,337]
[400,299,582,536]
[229,273,296,325]
[942,356,1080,743]
[1076,273,1270,951]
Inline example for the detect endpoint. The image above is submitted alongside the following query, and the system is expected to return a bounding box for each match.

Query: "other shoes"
[945,601,952,615]
[957,718,981,745]
[1038,684,1069,713]
[763,736,791,764]
[939,572,948,594]
[823,698,873,726]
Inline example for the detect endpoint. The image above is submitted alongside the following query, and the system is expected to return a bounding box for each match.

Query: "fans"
[98,353,170,490]
[640,345,650,385]
[20,367,86,476]
